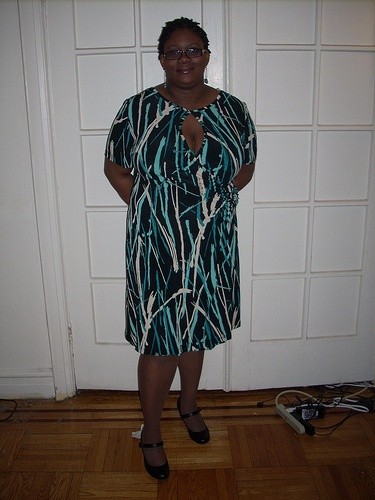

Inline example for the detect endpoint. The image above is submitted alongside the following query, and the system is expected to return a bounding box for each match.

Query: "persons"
[104,17,259,480]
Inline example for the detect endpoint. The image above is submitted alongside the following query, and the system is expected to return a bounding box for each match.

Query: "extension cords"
[277,405,306,435]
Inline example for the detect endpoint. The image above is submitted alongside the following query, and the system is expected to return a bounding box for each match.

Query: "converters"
[297,407,326,420]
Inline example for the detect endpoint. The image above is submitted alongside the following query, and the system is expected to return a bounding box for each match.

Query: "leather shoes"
[174,394,211,445]
[139,427,171,479]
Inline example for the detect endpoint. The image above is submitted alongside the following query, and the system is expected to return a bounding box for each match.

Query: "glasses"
[159,48,211,61]
